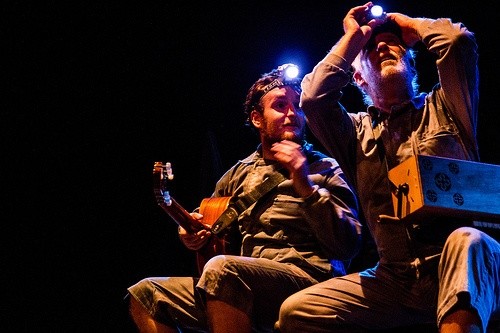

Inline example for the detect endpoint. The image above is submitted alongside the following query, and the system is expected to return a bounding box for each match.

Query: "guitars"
[153,162,238,277]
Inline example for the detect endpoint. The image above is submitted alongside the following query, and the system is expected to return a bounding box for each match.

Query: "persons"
[120,69,363,333]
[278,1,500,333]
